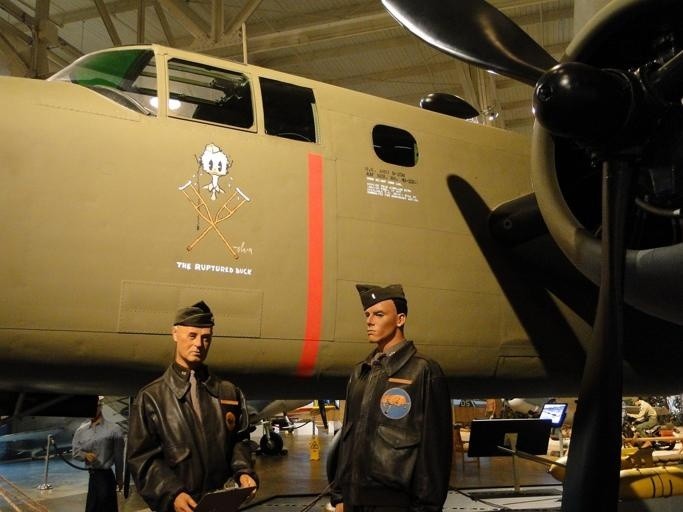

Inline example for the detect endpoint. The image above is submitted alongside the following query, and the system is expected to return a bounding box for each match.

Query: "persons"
[329,283,452,512]
[71,399,124,512]
[126,300,260,512]
[626,396,658,449]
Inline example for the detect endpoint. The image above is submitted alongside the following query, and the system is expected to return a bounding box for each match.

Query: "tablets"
[194,485,257,512]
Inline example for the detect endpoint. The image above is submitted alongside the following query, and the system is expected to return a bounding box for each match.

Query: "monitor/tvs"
[539,403,568,426]
[468,418,552,455]
